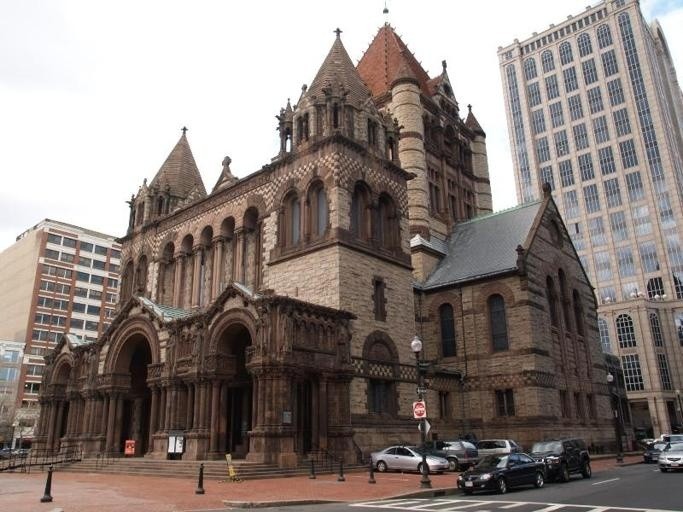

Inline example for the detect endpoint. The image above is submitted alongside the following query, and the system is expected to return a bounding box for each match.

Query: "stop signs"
[413,401,427,418]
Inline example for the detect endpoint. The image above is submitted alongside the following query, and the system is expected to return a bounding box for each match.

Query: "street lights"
[675,389,683,419]
[410,336,433,489]
[606,372,624,463]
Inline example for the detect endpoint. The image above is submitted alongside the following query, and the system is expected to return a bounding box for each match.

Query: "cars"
[639,433,683,473]
[0,448,28,459]
[370,438,591,494]
